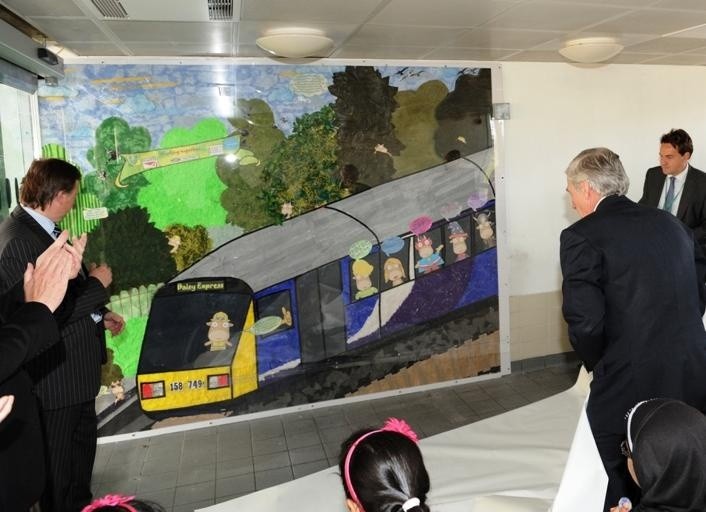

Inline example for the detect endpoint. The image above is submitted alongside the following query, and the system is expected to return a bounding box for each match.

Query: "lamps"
[255,27,335,65]
[559,35,623,64]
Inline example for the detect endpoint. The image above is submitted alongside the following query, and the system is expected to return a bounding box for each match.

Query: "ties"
[662,176,676,213]
[51,222,87,280]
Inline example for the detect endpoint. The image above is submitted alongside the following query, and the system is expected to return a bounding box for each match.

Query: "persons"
[559,147,705,512]
[0,394,15,424]
[0,227,87,512]
[606,397,706,512]
[0,157,124,512]
[638,129,706,319]
[82,494,164,512]
[337,418,429,512]
